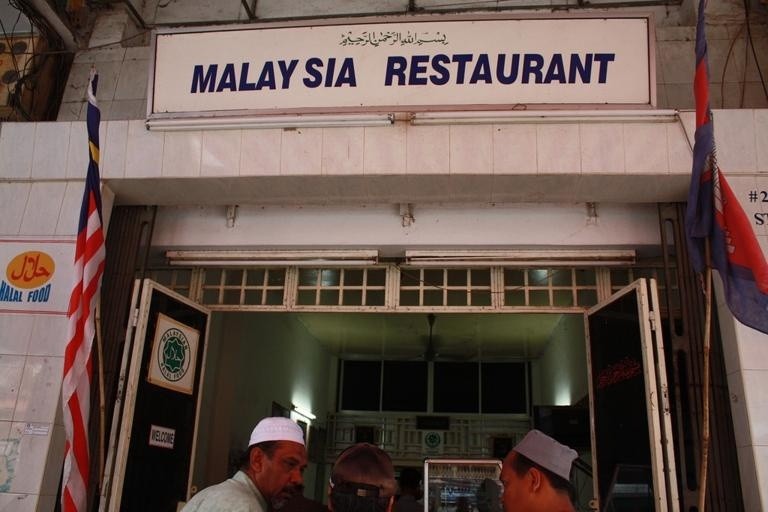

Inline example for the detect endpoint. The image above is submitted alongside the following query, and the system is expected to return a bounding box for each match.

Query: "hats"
[247,416,306,449]
[329,443,398,498]
[512,427,579,481]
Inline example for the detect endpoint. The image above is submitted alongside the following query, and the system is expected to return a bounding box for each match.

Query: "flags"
[685,0,767,334]
[60,69,106,512]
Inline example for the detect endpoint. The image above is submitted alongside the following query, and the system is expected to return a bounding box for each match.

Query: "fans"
[401,313,472,360]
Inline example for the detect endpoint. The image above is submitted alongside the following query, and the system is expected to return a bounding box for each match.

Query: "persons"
[178,417,309,512]
[326,428,592,512]
